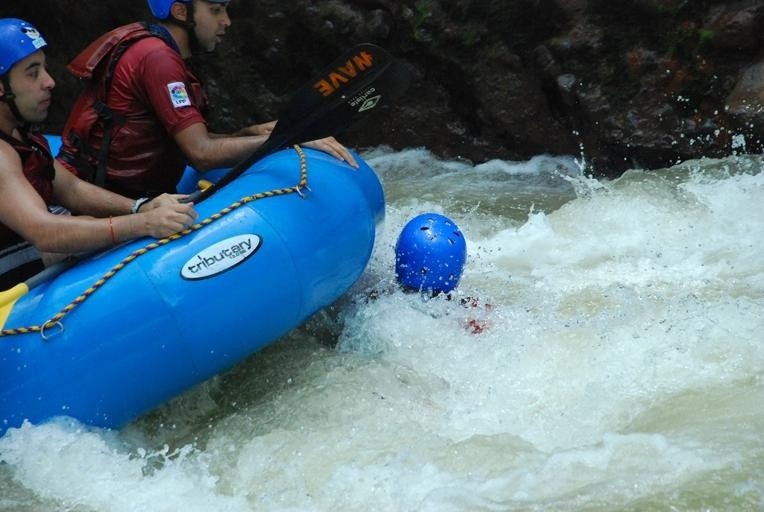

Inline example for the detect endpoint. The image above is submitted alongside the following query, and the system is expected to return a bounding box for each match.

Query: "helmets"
[148,0,174,20]
[0,18,48,75]
[395,212,466,294]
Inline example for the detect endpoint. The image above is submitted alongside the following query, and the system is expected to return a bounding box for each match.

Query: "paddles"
[0,179,214,331]
[188,43,410,204]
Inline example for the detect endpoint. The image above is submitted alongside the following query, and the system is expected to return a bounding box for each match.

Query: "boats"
[0,142,385,431]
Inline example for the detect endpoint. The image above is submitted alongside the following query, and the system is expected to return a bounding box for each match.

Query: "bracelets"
[129,197,150,214]
[108,215,115,247]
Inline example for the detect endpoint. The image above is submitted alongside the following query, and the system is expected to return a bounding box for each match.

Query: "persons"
[350,212,493,336]
[52,1,361,201]
[0,16,199,291]
